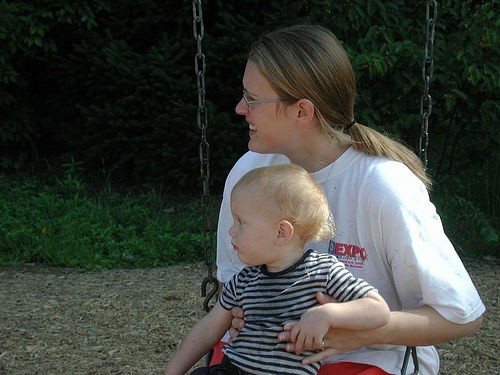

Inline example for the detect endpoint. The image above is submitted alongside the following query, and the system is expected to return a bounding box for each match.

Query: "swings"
[188,0,442,375]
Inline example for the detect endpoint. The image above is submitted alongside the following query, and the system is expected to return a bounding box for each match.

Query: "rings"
[321,338,325,352]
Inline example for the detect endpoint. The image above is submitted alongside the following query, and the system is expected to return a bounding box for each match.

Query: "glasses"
[242,90,298,108]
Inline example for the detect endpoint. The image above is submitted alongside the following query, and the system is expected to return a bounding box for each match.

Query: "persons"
[207,24,487,375]
[163,163,390,375]
